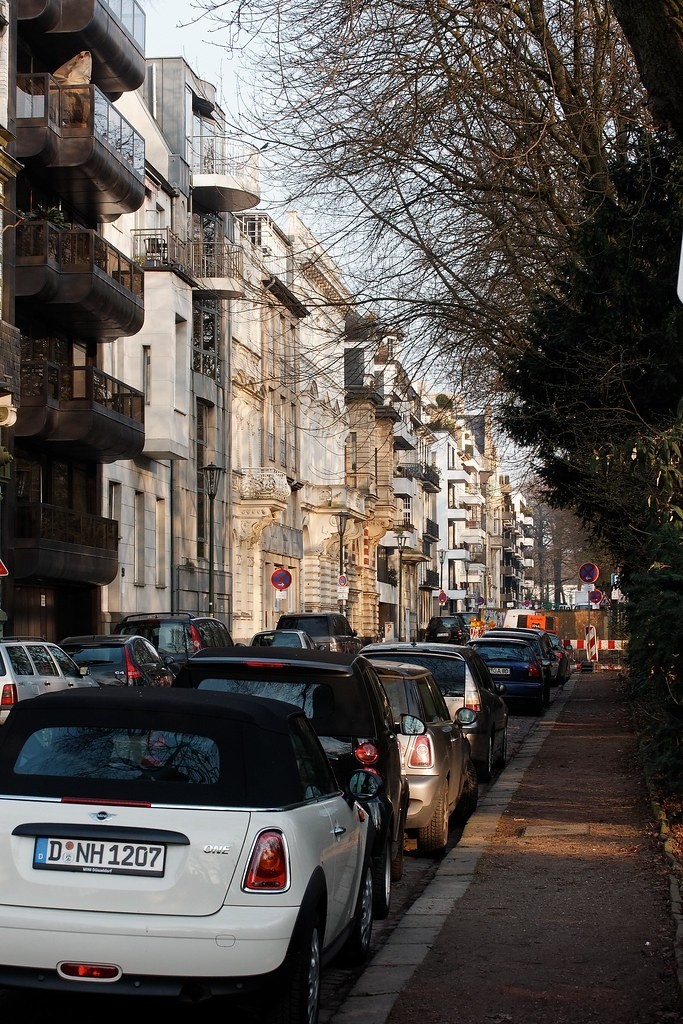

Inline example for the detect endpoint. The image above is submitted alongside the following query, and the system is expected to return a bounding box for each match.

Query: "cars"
[249,629,326,653]
[53,635,177,688]
[357,641,509,784]
[0,636,100,731]
[480,627,572,687]
[167,646,410,924]
[0,685,389,1024]
[463,637,552,717]
[367,658,480,859]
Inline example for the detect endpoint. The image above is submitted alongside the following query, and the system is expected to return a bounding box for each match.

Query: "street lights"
[434,548,448,640]
[464,556,472,612]
[199,460,224,620]
[334,509,350,614]
[393,531,407,643]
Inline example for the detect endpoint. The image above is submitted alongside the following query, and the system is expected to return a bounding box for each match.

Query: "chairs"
[51,735,114,770]
[144,237,166,264]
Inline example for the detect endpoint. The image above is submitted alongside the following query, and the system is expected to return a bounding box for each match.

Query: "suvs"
[112,611,249,688]
[275,612,363,655]
